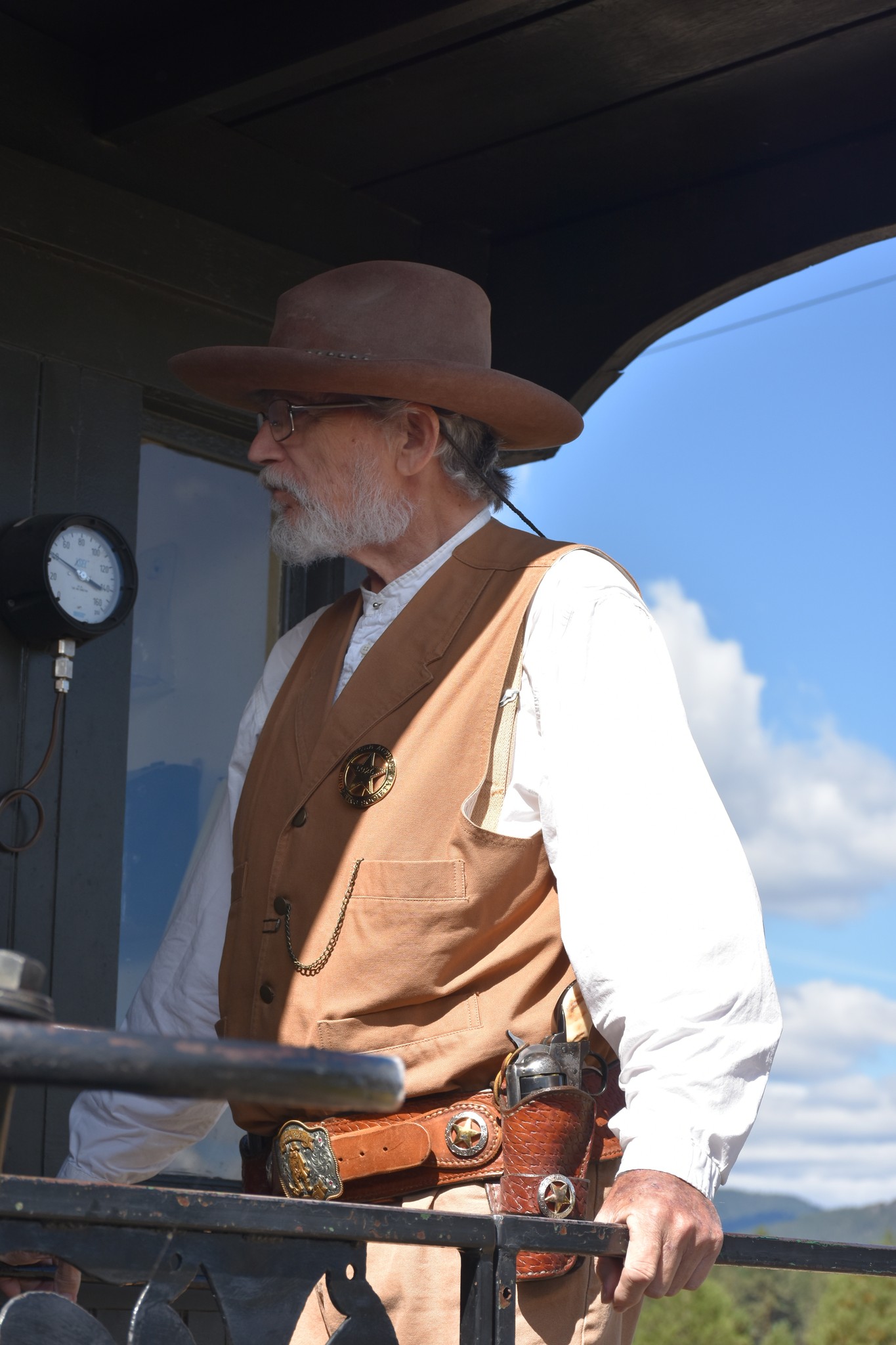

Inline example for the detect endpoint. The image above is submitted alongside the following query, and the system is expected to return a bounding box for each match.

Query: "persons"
[47,282,784,1345]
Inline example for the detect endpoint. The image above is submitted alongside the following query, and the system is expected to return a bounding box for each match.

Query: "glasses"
[255,399,370,443]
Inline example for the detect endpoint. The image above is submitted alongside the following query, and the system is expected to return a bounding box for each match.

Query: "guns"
[505,977,610,1284]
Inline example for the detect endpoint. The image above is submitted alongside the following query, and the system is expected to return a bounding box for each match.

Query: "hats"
[166,259,585,452]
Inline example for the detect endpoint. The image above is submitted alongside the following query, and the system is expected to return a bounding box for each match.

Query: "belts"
[238,1066,625,1207]
